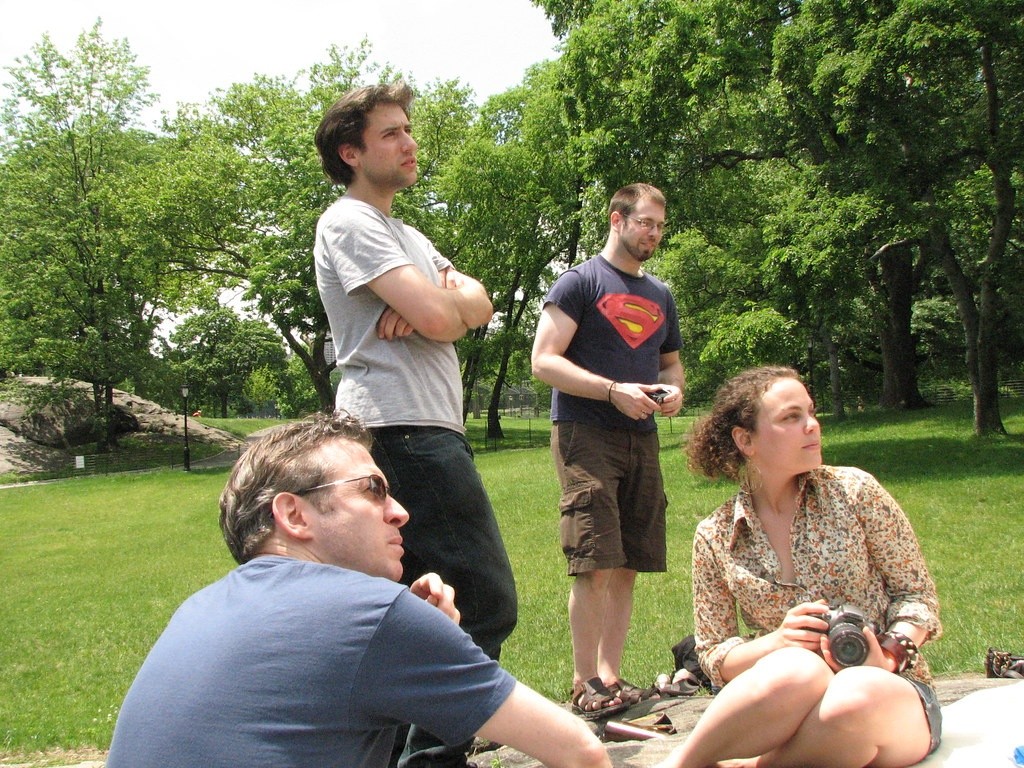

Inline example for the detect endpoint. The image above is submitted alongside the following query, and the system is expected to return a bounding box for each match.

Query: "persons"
[656,363,945,768]
[313,79,520,768]
[530,181,687,719]
[104,410,614,768]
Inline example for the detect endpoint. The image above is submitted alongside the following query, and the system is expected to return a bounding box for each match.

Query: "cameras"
[807,604,874,667]
[645,390,672,405]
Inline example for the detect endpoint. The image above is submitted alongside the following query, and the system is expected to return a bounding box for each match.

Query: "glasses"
[268,474,390,518]
[621,213,668,235]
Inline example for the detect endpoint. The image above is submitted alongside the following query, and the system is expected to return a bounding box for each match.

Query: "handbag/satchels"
[671,634,710,688]
[987,646,1023,680]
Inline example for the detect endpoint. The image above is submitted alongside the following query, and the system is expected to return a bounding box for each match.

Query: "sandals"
[570,677,630,721]
[606,679,663,703]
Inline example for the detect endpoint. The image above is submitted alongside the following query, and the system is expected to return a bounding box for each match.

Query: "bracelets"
[876,631,918,675]
[608,380,620,403]
